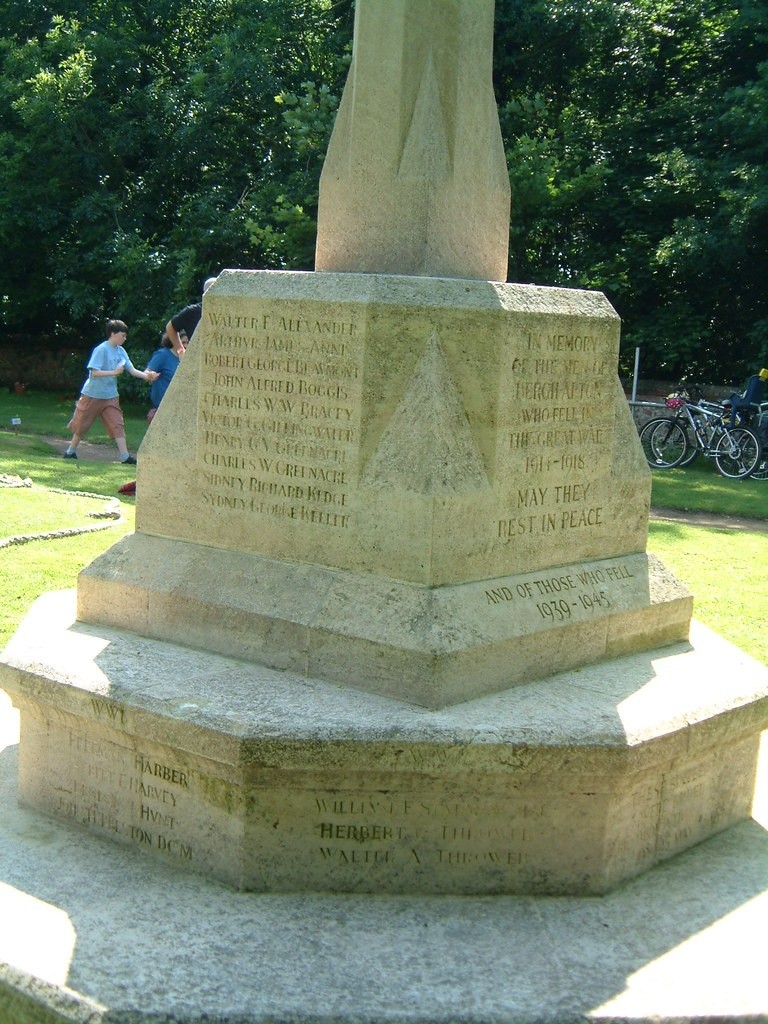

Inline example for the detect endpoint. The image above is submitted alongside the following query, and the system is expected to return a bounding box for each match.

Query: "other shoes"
[121,456,137,465]
[64,452,77,460]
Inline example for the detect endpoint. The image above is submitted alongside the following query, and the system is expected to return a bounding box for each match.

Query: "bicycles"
[638,383,768,481]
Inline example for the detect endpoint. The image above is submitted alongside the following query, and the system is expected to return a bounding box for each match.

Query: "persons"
[143,277,218,423]
[64,320,159,465]
